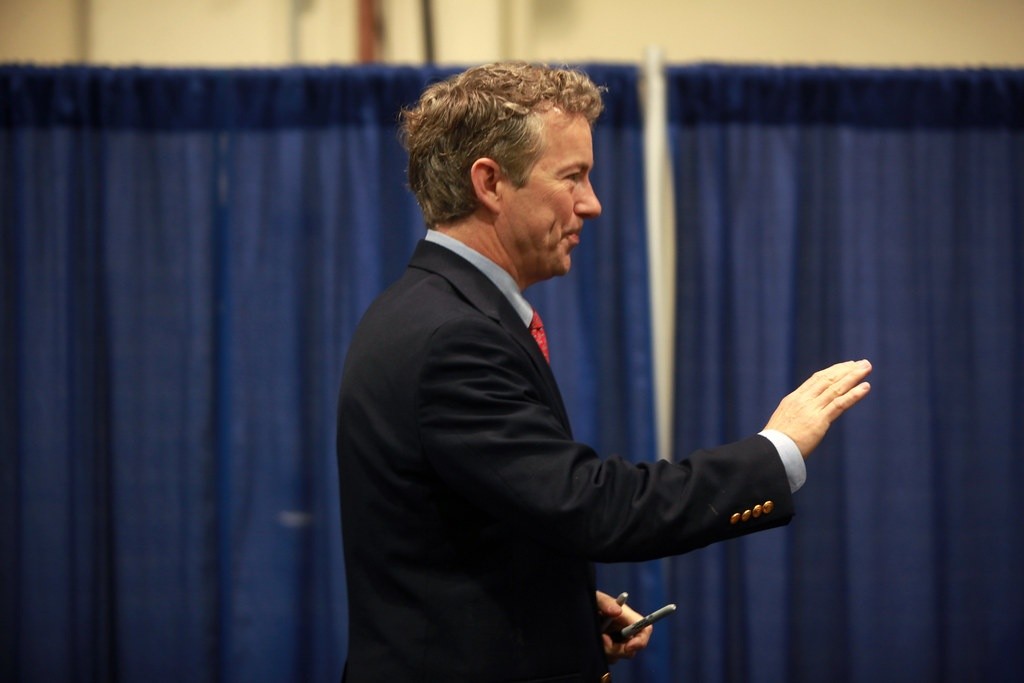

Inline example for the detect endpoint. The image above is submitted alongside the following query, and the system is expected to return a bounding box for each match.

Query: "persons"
[336,60,872,683]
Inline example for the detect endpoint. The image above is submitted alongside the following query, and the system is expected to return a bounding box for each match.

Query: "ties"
[528,307,552,365]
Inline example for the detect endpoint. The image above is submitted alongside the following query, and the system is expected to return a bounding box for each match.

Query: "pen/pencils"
[601,590,677,642]
